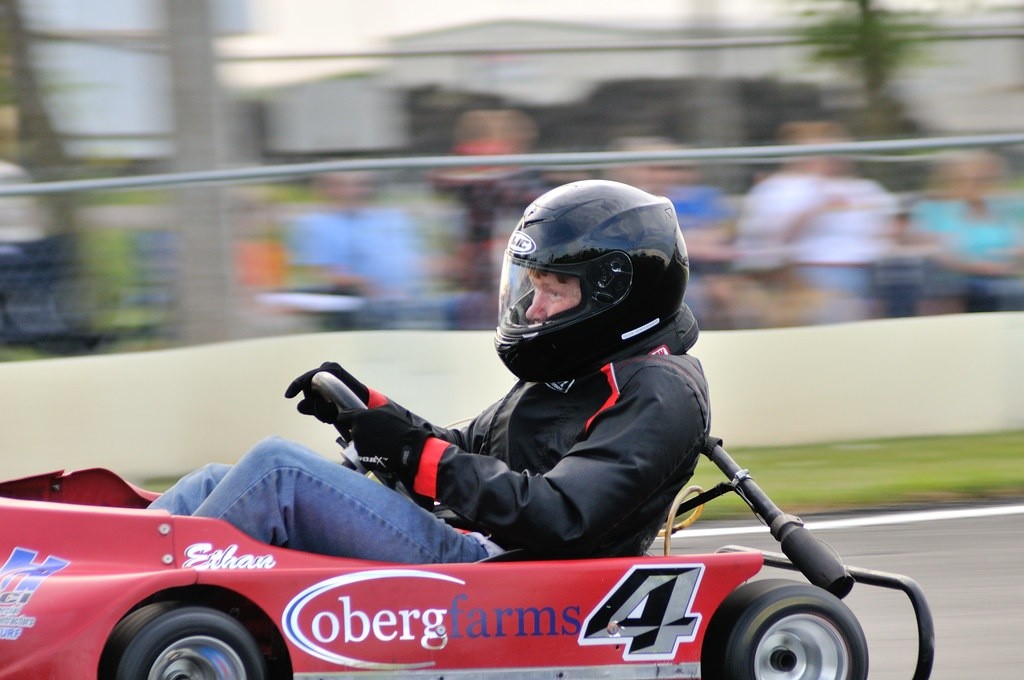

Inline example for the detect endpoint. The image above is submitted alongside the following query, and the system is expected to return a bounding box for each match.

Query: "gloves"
[285,362,452,500]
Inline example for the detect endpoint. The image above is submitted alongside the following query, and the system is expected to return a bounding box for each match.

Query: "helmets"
[494,179,689,378]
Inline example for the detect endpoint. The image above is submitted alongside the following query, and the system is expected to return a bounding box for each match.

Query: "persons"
[145,179,712,565]
[285,109,1024,334]
